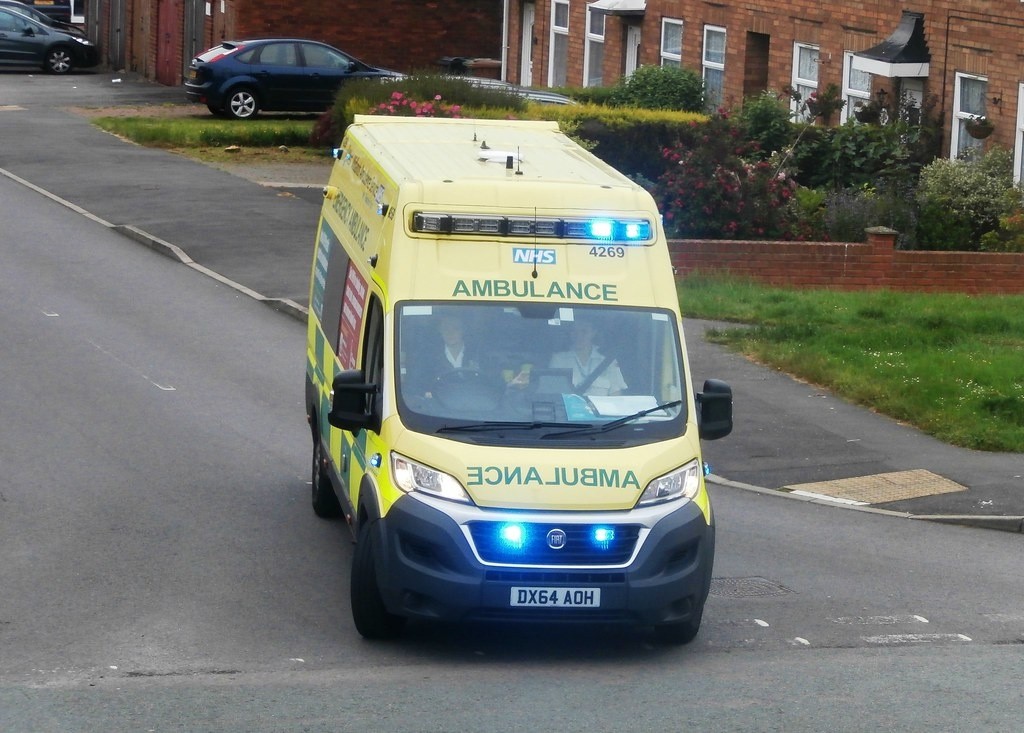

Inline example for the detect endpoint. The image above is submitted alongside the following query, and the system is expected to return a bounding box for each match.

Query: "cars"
[472,84,580,108]
[0,6,100,75]
[363,76,514,88]
[0,0,87,39]
[184,37,409,120]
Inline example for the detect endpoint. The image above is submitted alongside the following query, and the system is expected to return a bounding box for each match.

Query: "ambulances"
[304,114,734,650]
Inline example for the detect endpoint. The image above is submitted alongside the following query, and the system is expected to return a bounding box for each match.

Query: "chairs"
[276,50,297,66]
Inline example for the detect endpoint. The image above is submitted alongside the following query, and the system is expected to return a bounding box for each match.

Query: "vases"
[968,126,992,139]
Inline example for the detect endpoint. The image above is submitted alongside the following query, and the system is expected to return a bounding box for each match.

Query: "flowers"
[963,115,993,126]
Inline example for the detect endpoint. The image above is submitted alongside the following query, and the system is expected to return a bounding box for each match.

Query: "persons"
[548,316,627,396]
[416,311,505,390]
